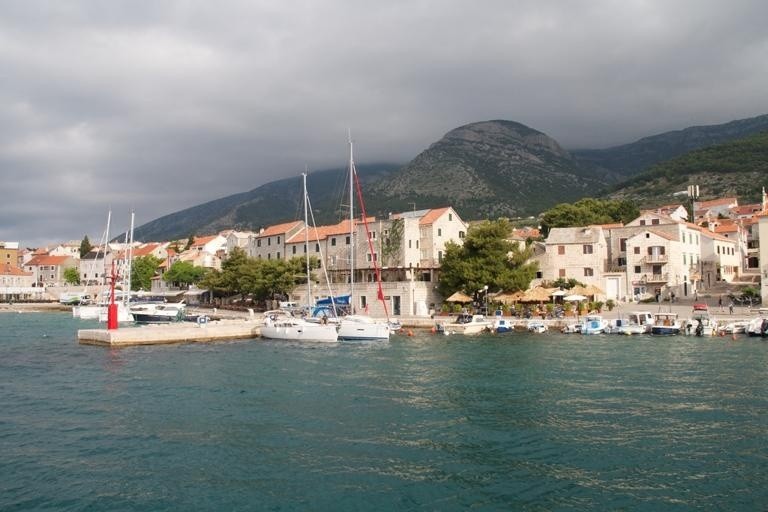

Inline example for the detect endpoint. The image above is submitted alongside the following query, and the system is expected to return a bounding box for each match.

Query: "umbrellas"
[487,283,607,318]
[444,290,475,302]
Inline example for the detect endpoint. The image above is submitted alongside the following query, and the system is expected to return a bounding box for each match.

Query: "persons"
[728,300,735,315]
[718,295,726,312]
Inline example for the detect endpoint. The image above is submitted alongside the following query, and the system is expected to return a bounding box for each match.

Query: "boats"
[561,301,765,338]
[443,312,549,336]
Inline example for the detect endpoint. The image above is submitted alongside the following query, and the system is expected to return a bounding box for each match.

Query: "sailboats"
[250,132,405,347]
[58,202,184,327]
